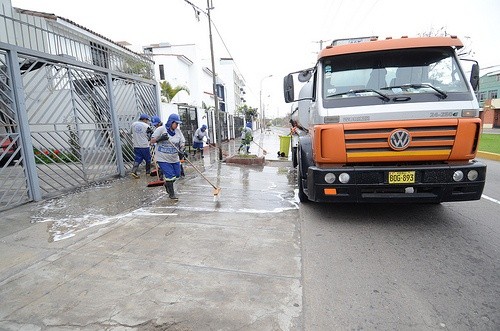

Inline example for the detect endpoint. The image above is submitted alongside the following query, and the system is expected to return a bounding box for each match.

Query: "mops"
[251,140,267,155]
[202,139,222,160]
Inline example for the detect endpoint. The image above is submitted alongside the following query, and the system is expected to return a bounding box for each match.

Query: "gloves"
[179,149,189,159]
[155,133,169,144]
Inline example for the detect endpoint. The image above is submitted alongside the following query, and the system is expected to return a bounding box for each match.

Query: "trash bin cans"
[278,136,290,157]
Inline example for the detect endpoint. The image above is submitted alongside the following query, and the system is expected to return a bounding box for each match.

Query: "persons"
[127,114,154,178]
[151,116,185,177]
[237,121,254,153]
[193,125,211,159]
[366,67,405,89]
[150,113,192,200]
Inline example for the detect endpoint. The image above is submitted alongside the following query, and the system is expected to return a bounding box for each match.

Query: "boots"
[131,162,140,179]
[200,150,204,159]
[237,144,251,153]
[180,164,185,177]
[146,163,152,176]
[192,150,197,158]
[163,181,178,200]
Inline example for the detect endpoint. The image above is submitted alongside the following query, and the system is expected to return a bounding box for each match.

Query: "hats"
[200,124,208,132]
[151,116,160,126]
[246,122,252,128]
[165,114,182,136]
[140,114,151,121]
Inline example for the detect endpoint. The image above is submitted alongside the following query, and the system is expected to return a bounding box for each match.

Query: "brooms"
[150,146,159,177]
[146,139,164,188]
[166,138,222,196]
